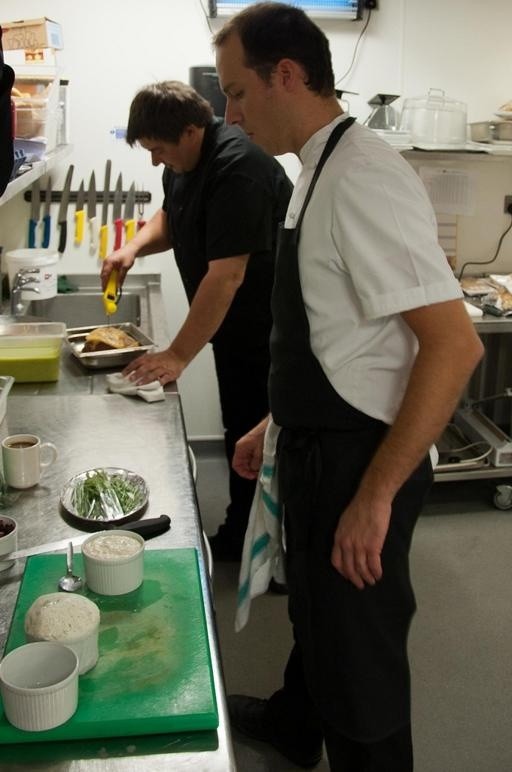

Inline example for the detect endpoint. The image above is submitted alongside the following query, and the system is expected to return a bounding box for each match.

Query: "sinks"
[24,293,140,329]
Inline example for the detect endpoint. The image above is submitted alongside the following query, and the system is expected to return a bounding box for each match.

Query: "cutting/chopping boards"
[0,548,221,745]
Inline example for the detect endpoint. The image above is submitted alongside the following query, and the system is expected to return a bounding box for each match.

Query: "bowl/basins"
[0,641,81,732]
[0,515,19,572]
[24,591,102,676]
[82,528,147,596]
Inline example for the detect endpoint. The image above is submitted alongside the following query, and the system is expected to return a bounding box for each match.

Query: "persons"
[210,3,492,772]
[99,79,293,563]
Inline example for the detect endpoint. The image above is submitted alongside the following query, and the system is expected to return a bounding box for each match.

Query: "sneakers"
[227,694,323,770]
[207,529,291,596]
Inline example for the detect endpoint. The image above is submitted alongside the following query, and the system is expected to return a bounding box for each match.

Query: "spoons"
[59,541,82,592]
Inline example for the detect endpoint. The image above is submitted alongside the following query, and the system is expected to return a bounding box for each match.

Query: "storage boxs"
[1,16,64,51]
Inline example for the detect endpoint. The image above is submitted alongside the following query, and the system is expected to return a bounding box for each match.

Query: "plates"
[59,464,150,524]
[369,128,415,156]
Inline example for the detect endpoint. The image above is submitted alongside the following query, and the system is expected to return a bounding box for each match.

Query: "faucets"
[13,266,39,285]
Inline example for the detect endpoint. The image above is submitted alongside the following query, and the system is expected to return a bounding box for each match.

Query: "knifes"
[75,179,86,244]
[124,181,136,246]
[113,172,124,252]
[28,181,42,249]
[0,514,171,563]
[42,176,53,249]
[99,159,112,259]
[57,164,74,253]
[87,170,99,250]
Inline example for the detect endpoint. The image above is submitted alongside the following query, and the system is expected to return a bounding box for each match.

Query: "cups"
[2,433,58,491]
